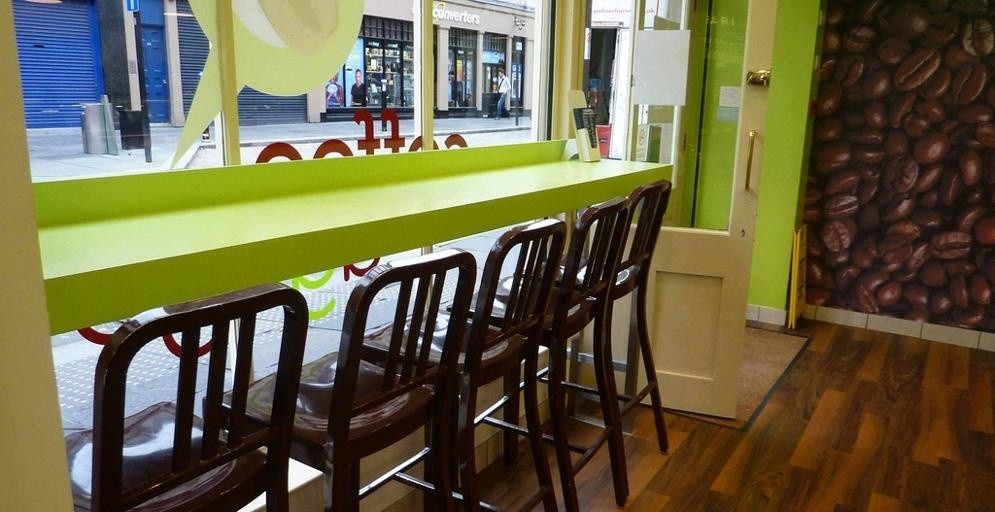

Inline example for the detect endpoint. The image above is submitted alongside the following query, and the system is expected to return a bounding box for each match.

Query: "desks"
[32,139,673,337]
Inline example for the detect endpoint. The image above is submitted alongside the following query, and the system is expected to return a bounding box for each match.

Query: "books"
[575,107,602,162]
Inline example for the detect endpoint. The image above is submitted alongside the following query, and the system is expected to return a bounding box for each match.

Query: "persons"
[351,69,367,106]
[494,68,512,120]
[325,73,344,107]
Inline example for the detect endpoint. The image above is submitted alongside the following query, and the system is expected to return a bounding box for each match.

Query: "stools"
[362,216,570,509]
[64,281,309,512]
[522,180,672,495]
[446,195,633,508]
[204,248,478,512]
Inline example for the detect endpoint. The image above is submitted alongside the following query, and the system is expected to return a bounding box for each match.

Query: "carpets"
[661,325,813,432]
